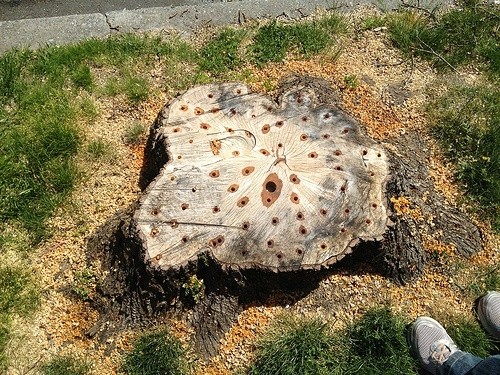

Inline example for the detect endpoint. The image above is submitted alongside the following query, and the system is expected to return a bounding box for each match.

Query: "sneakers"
[474,289,500,338]
[407,312,459,375]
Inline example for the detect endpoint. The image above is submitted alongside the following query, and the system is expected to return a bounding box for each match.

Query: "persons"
[408,290,500,374]
